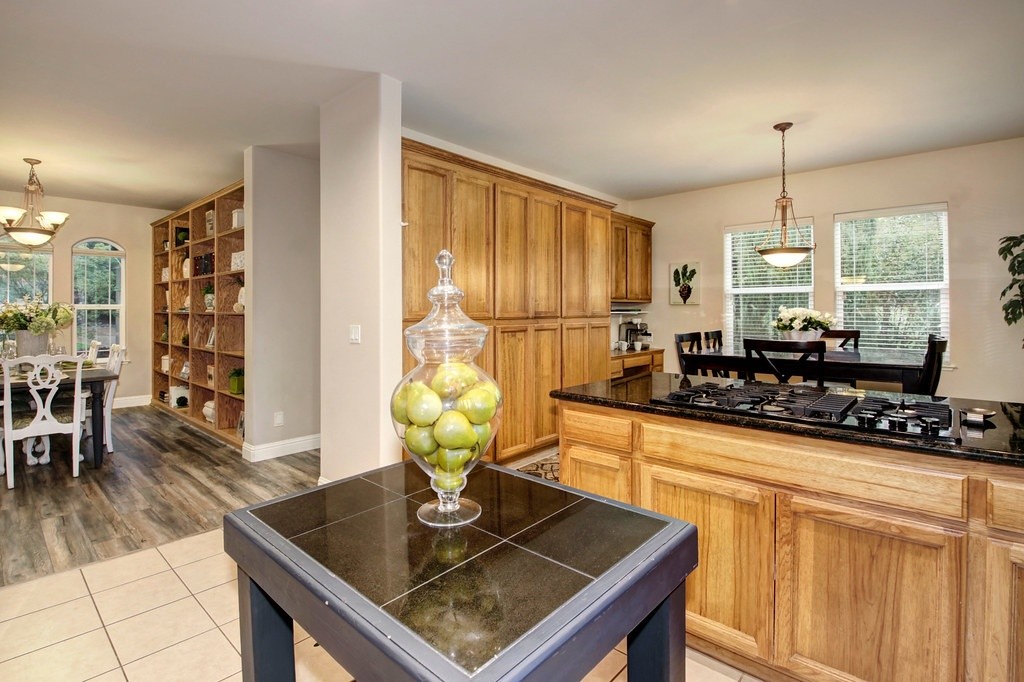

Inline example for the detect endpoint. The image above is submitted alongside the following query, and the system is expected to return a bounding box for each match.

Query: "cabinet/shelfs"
[149,178,245,451]
[400,148,664,462]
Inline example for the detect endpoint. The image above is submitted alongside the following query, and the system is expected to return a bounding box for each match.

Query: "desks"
[0,364,119,470]
[222,457,699,682]
[681,343,926,394]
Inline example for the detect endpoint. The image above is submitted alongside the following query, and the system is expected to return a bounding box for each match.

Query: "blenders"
[618,319,652,349]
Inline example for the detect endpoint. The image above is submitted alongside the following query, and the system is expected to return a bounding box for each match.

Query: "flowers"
[769,304,840,332]
[0,291,75,348]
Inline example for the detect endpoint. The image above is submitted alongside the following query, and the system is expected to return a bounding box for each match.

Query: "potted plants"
[227,366,244,395]
[201,283,214,310]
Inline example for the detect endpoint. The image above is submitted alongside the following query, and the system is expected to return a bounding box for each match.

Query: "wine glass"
[2,342,66,377]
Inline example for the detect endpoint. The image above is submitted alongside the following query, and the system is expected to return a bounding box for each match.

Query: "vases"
[781,329,824,342]
[15,330,49,371]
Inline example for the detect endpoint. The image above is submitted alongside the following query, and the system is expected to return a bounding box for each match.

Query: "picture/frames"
[669,261,701,306]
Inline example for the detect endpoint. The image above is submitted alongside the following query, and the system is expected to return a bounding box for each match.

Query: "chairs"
[917,330,947,395]
[0,340,126,489]
[742,338,826,388]
[674,330,728,378]
[802,329,861,388]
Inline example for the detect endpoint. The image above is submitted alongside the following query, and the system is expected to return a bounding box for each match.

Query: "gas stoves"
[648,378,957,444]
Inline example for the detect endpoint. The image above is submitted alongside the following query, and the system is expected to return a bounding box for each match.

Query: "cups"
[610,341,615,351]
[634,342,642,351]
[621,342,630,351]
[619,341,625,350]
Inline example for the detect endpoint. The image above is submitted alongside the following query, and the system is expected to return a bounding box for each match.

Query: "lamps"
[754,123,816,270]
[0,158,69,248]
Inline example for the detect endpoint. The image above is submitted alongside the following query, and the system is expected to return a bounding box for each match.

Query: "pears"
[393,362,499,489]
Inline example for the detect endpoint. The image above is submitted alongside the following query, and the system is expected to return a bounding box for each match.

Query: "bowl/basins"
[959,407,997,424]
